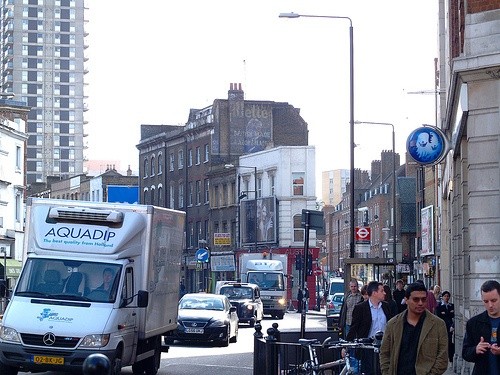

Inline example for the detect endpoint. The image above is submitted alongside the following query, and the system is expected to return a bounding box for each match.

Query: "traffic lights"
[307,254,315,276]
[294,254,301,272]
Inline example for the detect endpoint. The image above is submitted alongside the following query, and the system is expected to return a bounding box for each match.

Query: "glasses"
[408,296,427,303]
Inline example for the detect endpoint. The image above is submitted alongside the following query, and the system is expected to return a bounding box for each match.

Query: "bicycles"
[283,336,371,375]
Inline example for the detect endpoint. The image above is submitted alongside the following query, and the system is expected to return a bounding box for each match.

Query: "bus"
[325,278,365,314]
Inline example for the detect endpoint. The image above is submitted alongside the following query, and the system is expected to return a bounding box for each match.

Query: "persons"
[433,285,441,301]
[415,280,438,313]
[180,282,185,299]
[434,291,455,368]
[360,284,369,302]
[393,279,407,315]
[338,278,365,375]
[383,284,398,319]
[91,268,115,295]
[378,283,449,375]
[341,280,395,375]
[462,280,500,375]
[296,288,309,313]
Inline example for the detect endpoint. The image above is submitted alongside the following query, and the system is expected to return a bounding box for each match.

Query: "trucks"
[1,197,186,375]
[241,260,292,319]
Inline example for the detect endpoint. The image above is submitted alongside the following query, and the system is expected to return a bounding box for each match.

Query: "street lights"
[279,12,364,297]
[351,119,396,287]
[224,164,259,254]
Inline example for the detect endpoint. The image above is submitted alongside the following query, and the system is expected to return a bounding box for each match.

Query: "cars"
[212,282,263,327]
[324,292,351,331]
[163,291,238,347]
